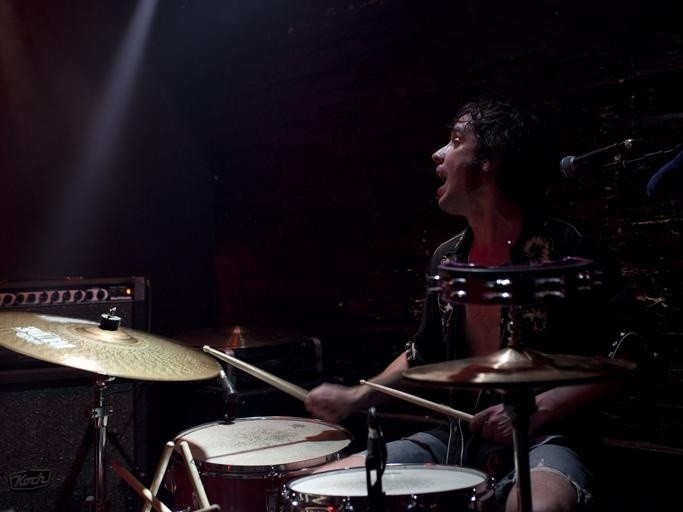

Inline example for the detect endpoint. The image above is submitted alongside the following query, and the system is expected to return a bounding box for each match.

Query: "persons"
[305,102,615,511]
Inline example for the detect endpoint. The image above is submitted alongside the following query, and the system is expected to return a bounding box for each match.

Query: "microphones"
[559,139,634,181]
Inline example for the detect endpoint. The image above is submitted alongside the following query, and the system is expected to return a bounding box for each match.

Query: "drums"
[165,415,354,512]
[278,460,497,512]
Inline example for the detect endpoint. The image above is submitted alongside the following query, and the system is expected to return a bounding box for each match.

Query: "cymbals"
[0,308,222,382]
[180,317,302,351]
[400,349,634,384]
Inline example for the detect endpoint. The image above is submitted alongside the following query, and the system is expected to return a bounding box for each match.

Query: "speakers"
[0,364,149,512]
